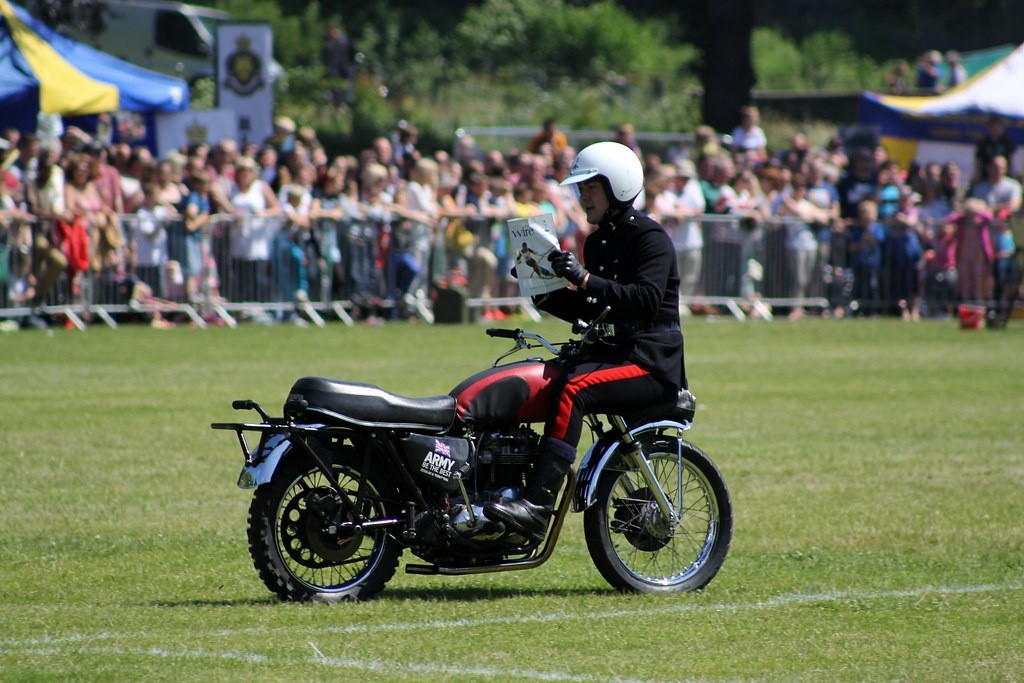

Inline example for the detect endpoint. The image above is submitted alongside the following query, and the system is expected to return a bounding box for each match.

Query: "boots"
[484,435,577,542]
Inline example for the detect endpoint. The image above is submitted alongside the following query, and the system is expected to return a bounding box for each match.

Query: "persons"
[0,105,1024,330]
[517,241,554,279]
[481,141,687,544]
[323,24,365,105]
[885,49,966,94]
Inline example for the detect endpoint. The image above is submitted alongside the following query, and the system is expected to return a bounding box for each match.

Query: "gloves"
[548,250,589,286]
[511,267,518,278]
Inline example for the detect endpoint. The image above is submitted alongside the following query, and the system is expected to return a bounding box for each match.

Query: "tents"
[0,0,189,130]
[864,43,1024,143]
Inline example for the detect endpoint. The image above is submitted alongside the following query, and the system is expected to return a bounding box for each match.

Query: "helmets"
[559,142,643,201]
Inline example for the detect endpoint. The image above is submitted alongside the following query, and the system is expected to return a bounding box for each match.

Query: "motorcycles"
[209,299,736,606]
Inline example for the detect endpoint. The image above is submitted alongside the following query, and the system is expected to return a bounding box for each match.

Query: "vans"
[52,0,286,103]
